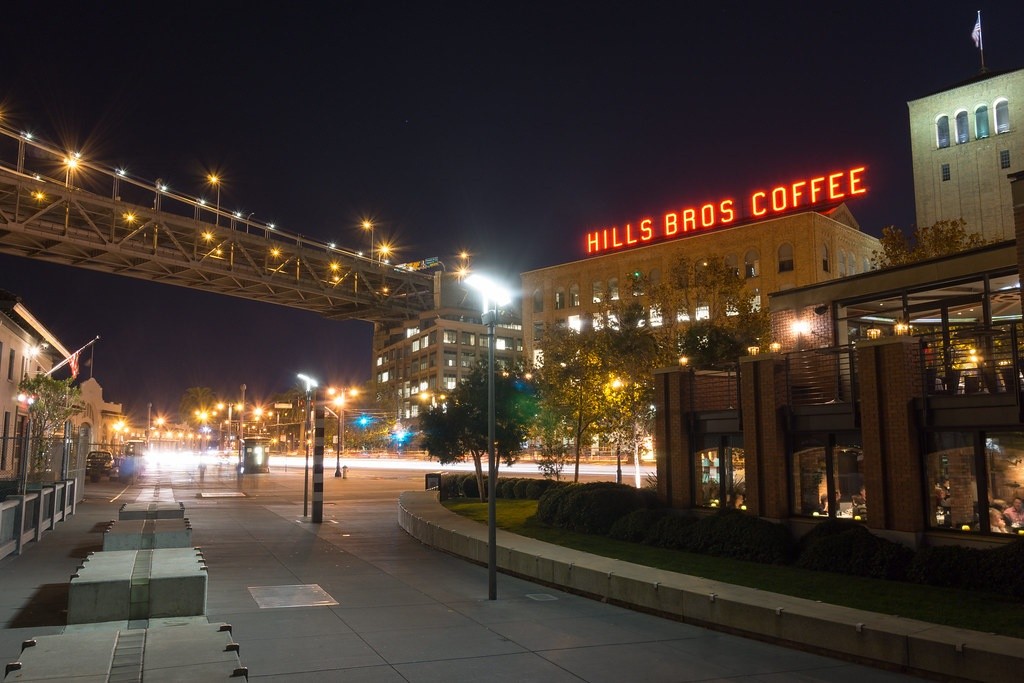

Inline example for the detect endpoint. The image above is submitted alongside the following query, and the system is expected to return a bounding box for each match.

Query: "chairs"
[925,367,1024,395]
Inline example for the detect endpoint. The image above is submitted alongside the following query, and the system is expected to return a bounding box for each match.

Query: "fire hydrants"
[342,464,349,479]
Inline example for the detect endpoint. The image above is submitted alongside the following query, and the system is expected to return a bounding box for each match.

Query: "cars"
[85,449,114,477]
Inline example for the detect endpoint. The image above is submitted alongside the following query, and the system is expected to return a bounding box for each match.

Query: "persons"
[702,478,1024,537]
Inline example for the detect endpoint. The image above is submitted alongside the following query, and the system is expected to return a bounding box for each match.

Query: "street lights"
[333,395,344,477]
[239,405,261,440]
[462,271,516,605]
[218,400,244,446]
